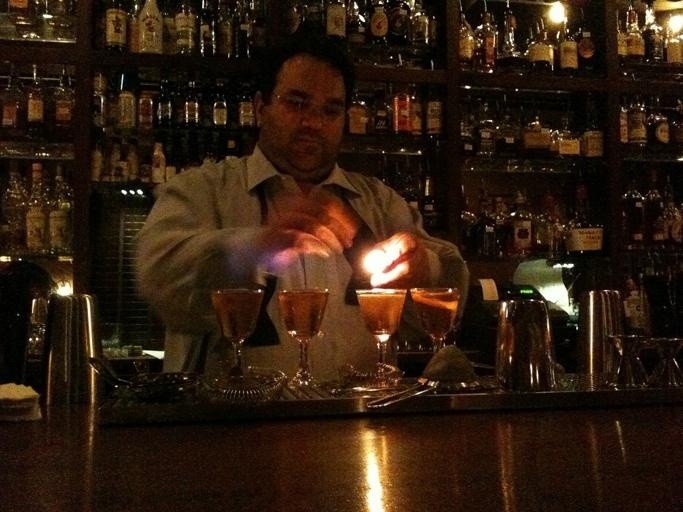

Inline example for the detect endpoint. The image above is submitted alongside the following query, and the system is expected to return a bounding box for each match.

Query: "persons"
[137,34,470,385]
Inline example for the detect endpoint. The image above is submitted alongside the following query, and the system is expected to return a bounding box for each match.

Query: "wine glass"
[277,286,334,394]
[355,283,406,390]
[212,286,266,387]
[410,285,461,351]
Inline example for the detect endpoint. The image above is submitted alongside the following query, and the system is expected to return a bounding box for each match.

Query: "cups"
[498,296,553,389]
[612,335,682,391]
[44,294,102,402]
[579,286,625,378]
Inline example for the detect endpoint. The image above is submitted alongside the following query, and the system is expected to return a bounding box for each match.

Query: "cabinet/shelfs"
[617,2,680,342]
[452,1,616,311]
[0,0,88,301]
[87,1,451,354]
